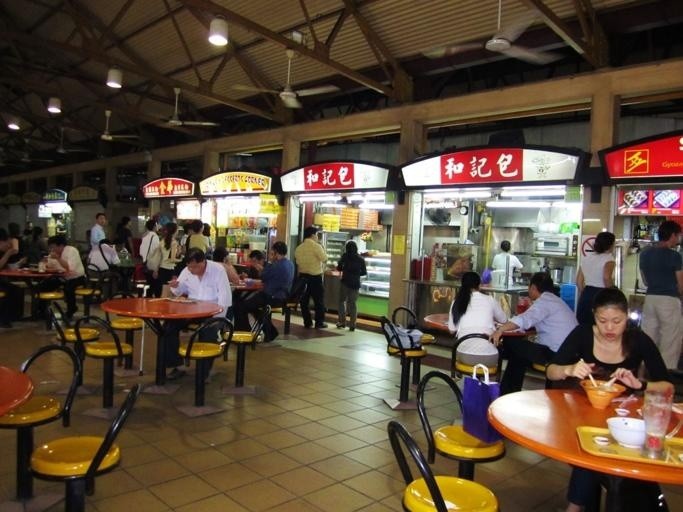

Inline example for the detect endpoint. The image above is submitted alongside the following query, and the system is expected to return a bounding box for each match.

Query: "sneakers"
[336,322,345,328]
[264,328,279,342]
[350,327,354,331]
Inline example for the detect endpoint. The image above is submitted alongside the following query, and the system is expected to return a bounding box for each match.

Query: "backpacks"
[383,323,424,350]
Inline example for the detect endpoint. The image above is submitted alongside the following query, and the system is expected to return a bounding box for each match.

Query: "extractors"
[492,208,541,229]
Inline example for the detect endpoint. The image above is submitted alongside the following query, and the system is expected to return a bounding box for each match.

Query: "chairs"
[387,421,499,512]
[75,315,133,420]
[380,316,419,410]
[221,305,271,393]
[103,292,147,377]
[30,383,142,512]
[452,333,503,384]
[0,288,13,329]
[532,361,554,389]
[417,371,506,480]
[278,277,308,335]
[46,301,99,384]
[34,276,66,337]
[74,264,103,319]
[393,307,437,391]
[174,316,234,418]
[0,343,83,497]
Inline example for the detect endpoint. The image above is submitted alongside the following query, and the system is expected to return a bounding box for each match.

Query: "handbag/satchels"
[109,264,120,278]
[133,263,148,282]
[174,257,187,278]
[462,364,504,442]
[140,261,153,276]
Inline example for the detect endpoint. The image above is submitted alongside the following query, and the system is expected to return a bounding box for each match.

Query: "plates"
[167,298,200,303]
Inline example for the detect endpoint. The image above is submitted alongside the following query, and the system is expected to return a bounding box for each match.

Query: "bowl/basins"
[607,416,645,450]
[8,263,20,272]
[593,436,609,446]
[615,408,629,416]
[579,377,626,410]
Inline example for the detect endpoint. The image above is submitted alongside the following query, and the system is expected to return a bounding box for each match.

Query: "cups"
[643,391,672,459]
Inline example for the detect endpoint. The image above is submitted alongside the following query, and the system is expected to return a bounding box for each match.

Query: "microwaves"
[533,233,572,256]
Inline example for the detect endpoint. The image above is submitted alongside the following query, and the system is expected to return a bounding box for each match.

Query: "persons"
[139,214,328,344]
[336,241,367,331]
[163,248,232,382]
[449,219,682,512]
[1,213,135,318]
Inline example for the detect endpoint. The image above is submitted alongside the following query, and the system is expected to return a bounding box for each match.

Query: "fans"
[424,0,564,66]
[231,50,340,110]
[129,87,221,134]
[4,152,56,164]
[69,110,140,141]
[36,127,88,154]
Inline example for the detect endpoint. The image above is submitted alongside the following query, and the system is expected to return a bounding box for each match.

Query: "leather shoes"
[65,306,77,319]
[305,323,313,328]
[166,368,185,379]
[315,324,328,327]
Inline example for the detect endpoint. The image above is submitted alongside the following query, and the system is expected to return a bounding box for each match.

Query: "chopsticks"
[581,358,598,387]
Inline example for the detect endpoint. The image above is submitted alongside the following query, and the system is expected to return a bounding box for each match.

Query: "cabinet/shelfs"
[317,231,348,271]
[356,250,391,321]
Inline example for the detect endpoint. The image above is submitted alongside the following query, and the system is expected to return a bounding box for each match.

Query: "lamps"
[46,96,61,113]
[208,15,228,46]
[105,65,123,89]
[7,112,21,130]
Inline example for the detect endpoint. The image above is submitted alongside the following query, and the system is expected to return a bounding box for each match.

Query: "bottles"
[39,262,44,273]
